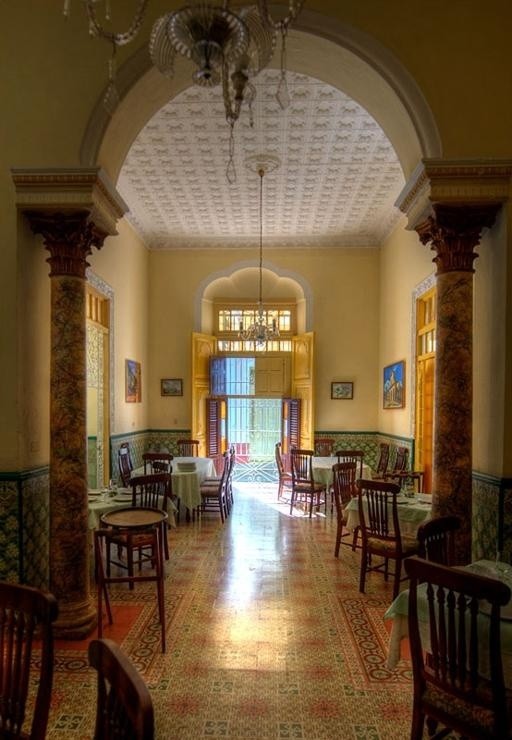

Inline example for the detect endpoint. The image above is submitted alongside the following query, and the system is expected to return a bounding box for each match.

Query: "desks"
[345,490,431,559]
[302,456,373,489]
[385,557,510,672]
[130,457,217,519]
[85,487,178,574]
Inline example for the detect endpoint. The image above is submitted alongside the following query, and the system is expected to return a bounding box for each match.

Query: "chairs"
[87,639,155,739]
[314,438,334,455]
[117,442,133,486]
[105,472,172,592]
[276,444,308,498]
[394,447,407,472]
[382,470,425,496]
[329,449,363,503]
[402,556,511,739]
[0,581,59,740]
[143,452,181,521]
[375,442,389,474]
[332,463,372,566]
[289,449,327,518]
[177,439,200,456]
[415,518,471,572]
[355,479,413,604]
[192,449,235,524]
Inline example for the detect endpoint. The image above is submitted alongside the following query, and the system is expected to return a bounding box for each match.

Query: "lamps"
[238,156,281,348]
[64,0,302,185]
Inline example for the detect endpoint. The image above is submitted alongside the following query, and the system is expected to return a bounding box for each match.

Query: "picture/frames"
[161,378,183,396]
[331,382,353,400]
[383,360,406,409]
[125,358,142,403]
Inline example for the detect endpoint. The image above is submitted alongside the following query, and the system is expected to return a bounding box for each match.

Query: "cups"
[109,478,117,492]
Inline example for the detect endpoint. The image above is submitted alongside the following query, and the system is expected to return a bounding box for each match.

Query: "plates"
[120,488,145,494]
[387,497,417,504]
[113,495,139,502]
